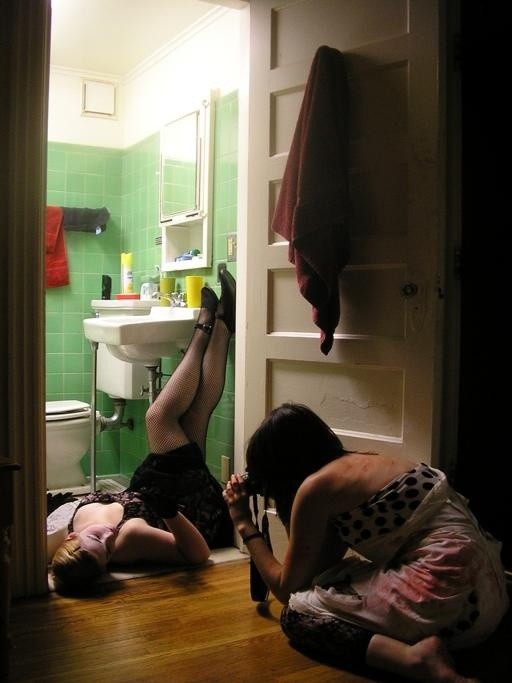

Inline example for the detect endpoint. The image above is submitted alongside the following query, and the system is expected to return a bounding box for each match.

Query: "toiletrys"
[101,273,112,299]
[141,277,152,302]
[101,274,112,301]
[140,274,153,299]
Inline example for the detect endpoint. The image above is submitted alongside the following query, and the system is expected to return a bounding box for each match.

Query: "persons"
[221,399,510,681]
[47,262,236,600]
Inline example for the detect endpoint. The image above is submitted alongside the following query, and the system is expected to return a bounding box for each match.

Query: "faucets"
[151,291,176,310]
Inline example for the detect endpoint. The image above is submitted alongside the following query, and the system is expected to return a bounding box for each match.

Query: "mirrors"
[159,110,201,224]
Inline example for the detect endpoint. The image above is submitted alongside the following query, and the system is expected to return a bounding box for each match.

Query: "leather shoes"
[194,287,218,335]
[213,268,235,332]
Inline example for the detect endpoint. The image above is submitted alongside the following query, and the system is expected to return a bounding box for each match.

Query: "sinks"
[81,304,203,359]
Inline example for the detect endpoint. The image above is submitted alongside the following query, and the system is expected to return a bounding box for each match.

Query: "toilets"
[46,341,153,489]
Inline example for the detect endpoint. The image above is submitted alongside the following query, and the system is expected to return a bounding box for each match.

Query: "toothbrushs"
[155,264,164,277]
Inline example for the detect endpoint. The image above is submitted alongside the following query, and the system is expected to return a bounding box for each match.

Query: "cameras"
[240,472,251,482]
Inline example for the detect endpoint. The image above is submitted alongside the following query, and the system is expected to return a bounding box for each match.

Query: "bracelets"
[241,530,264,545]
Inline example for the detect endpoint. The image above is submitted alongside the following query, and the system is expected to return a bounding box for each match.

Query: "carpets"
[47,479,250,591]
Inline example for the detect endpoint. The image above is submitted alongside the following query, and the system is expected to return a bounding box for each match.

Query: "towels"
[45,203,110,289]
[270,46,351,356]
